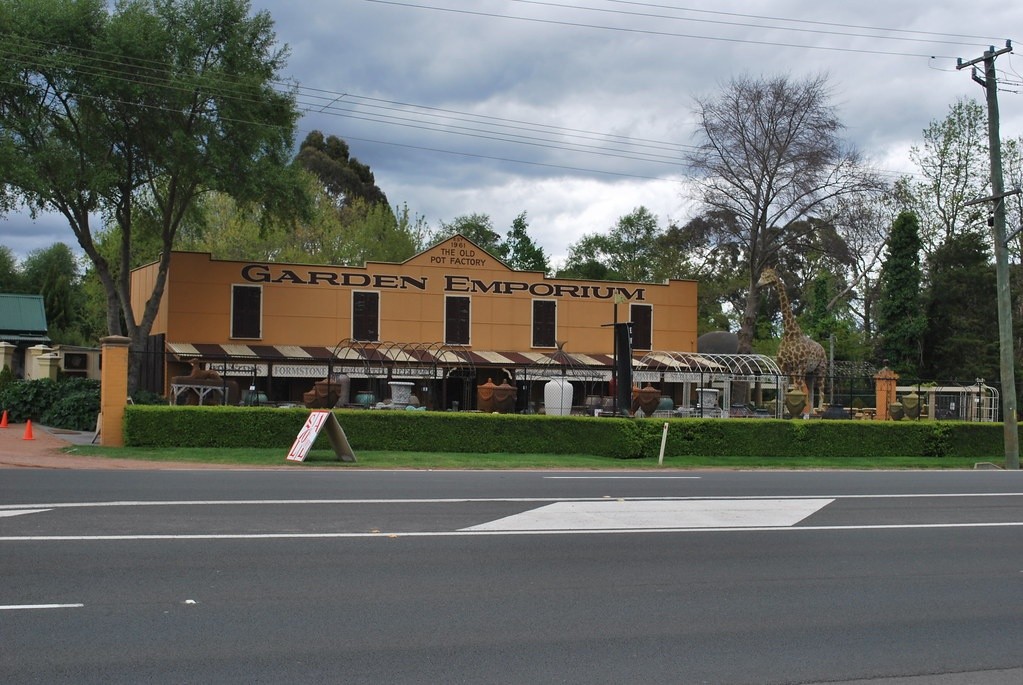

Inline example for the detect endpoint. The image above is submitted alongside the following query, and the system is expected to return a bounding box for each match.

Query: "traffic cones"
[0,410,10,428]
[22,419,35,440]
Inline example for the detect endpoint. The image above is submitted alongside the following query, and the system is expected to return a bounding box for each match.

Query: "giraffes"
[754,262,828,416]
